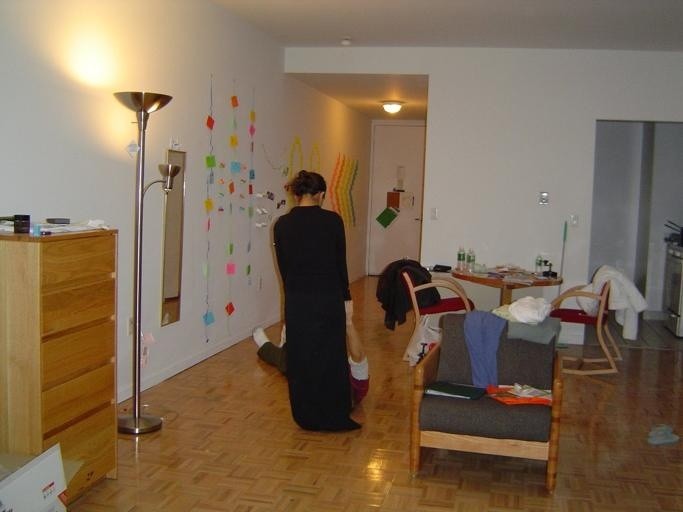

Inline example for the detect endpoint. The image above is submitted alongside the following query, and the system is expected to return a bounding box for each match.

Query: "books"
[425,380,492,405]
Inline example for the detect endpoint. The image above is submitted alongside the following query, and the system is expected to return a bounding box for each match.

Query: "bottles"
[466,248,474,273]
[535,253,542,275]
[456,246,465,273]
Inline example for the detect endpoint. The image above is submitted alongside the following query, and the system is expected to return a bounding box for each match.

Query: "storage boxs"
[0,438,90,511]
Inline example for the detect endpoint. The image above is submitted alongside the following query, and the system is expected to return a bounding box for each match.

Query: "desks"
[452,268,563,306]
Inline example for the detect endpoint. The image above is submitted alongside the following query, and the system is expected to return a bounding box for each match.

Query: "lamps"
[114,80,181,435]
[379,99,401,117]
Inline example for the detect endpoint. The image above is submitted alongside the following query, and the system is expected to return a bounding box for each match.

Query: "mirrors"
[160,148,187,325]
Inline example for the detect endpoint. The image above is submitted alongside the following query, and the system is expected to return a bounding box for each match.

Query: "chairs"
[547,265,632,376]
[402,266,476,325]
[407,312,566,497]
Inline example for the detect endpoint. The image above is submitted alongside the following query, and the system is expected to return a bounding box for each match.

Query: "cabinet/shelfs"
[0,226,115,502]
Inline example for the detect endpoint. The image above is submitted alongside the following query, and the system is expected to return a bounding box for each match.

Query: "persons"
[272,169,365,431]
[251,321,371,412]
